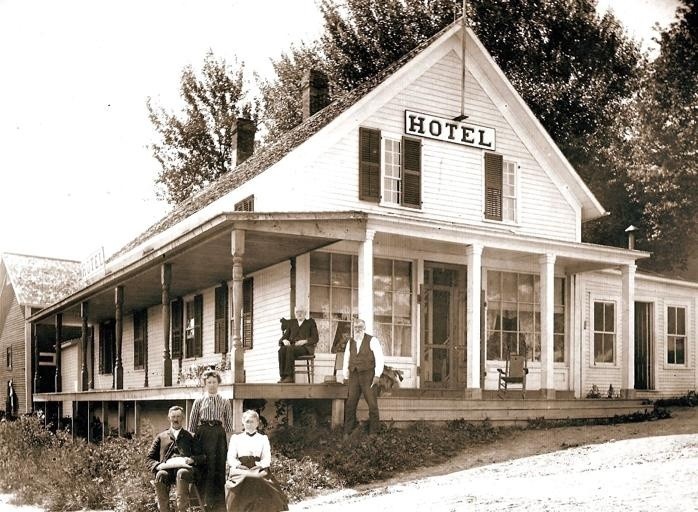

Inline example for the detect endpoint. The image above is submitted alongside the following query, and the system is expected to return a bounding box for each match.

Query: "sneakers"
[276,376,286,383]
[282,376,295,384]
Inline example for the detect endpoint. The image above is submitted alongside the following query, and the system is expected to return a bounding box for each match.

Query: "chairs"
[280,317,315,384]
[497,354,528,401]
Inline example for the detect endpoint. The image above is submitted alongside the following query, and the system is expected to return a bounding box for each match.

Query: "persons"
[225,409,284,512]
[188,371,233,495]
[277,304,318,383]
[144,405,205,512]
[343,319,384,439]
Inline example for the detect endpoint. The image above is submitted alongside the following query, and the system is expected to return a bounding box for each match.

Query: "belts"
[200,419,223,427]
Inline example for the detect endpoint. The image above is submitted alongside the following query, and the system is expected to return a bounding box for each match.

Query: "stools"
[167,481,205,512]
